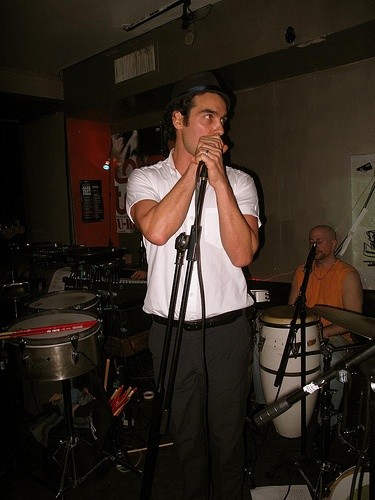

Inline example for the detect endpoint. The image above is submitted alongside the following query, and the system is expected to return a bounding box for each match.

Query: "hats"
[165,71,231,113]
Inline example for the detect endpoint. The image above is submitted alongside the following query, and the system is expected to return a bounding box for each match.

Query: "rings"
[206,150,208,156]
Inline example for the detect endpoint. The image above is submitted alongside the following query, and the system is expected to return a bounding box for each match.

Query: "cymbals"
[312,305,375,340]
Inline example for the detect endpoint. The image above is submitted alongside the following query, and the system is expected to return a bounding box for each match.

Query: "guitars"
[0,223,25,240]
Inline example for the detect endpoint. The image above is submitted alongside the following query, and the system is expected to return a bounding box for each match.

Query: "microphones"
[243,379,321,439]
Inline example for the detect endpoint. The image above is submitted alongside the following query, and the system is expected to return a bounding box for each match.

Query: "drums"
[25,288,103,313]
[324,463,369,499]
[257,304,325,439]
[5,309,104,382]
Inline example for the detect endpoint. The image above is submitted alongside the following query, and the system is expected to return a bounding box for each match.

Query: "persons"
[126,71,261,500]
[287,224,362,447]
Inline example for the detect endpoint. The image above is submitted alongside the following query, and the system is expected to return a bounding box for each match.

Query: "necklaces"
[313,257,337,280]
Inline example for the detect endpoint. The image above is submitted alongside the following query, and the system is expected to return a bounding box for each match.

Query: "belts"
[151,305,256,331]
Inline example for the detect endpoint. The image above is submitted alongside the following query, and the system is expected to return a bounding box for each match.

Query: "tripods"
[41,379,144,500]
